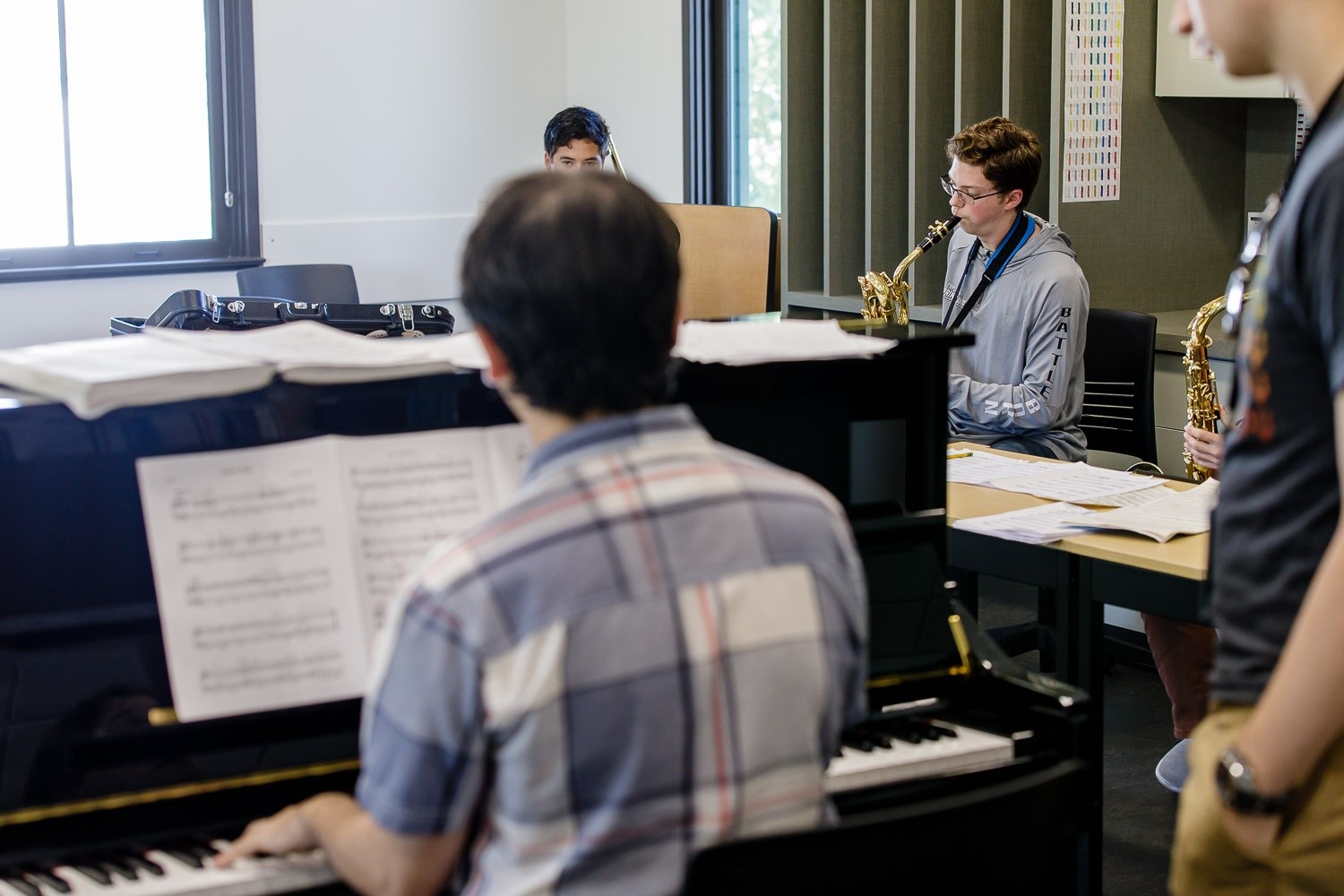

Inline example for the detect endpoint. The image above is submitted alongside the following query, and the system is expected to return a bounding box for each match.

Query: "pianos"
[0,312,1107,896]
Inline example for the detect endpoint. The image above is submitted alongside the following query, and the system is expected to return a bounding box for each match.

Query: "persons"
[218,169,870,896]
[544,107,609,173]
[1173,0,1344,896]
[940,116,1091,464]
[1141,402,1230,794]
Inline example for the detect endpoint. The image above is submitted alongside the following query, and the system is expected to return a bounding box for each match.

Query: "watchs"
[1218,750,1297,816]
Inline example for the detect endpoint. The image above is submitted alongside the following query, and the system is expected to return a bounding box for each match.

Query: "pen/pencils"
[947,452,973,460]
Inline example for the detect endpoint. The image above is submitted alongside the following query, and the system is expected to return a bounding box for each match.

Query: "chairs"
[1074,306,1160,471]
[239,262,360,306]
[662,202,777,334]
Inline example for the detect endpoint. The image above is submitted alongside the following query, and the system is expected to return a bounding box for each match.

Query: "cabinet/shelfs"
[1155,0,1291,99]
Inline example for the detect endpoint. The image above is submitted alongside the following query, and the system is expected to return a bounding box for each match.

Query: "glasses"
[939,172,1013,206]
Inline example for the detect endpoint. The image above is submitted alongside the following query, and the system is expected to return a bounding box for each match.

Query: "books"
[0,319,452,422]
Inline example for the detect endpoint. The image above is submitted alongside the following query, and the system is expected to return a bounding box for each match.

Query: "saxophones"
[857,216,963,326]
[1123,290,1255,481]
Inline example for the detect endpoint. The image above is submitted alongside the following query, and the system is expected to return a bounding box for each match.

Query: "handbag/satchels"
[110,291,455,338]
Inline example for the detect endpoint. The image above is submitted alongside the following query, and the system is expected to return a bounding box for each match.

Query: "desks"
[946,441,1214,722]
